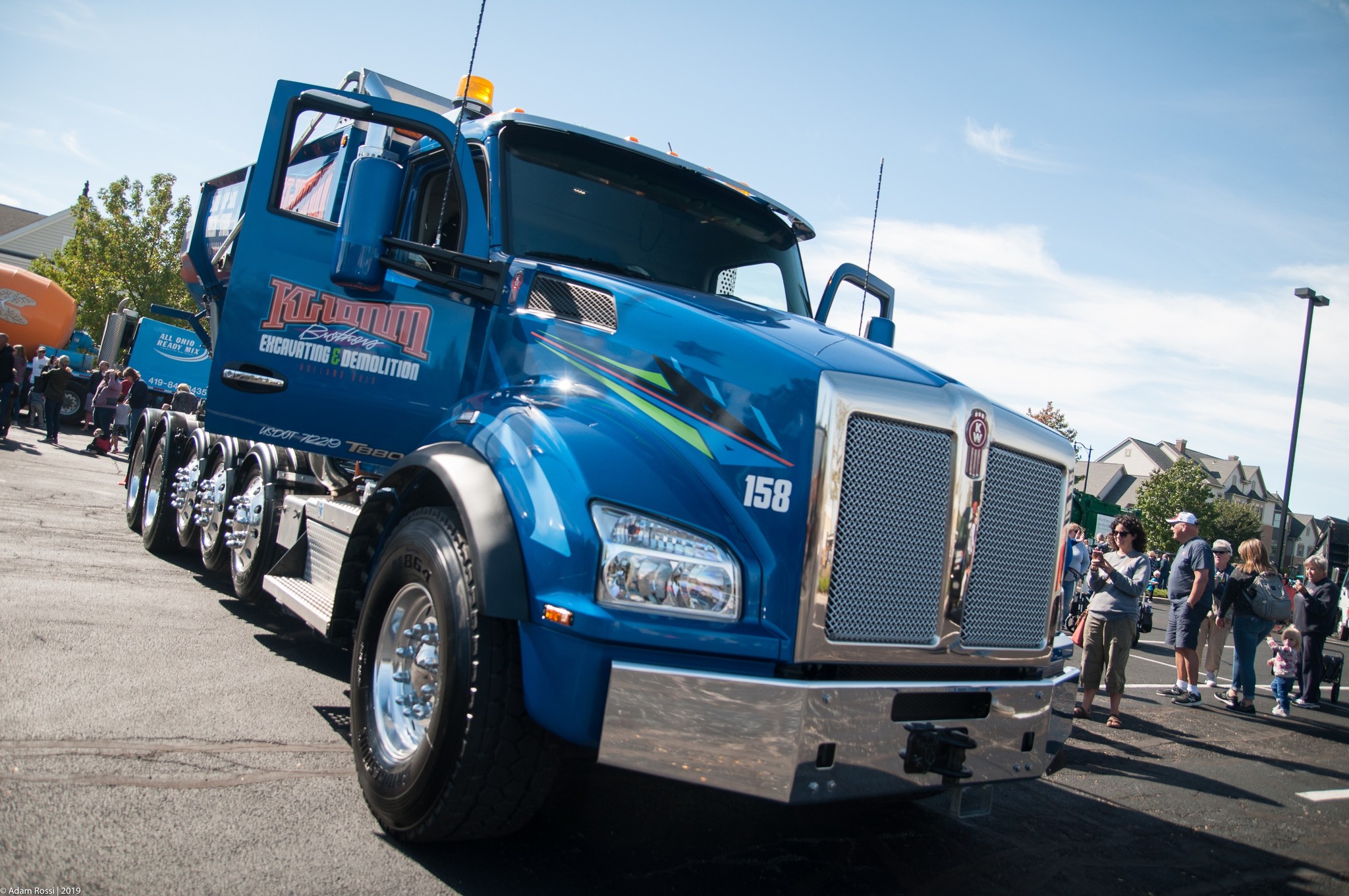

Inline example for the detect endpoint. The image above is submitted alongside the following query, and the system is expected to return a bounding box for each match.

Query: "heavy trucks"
[124,67,1087,856]
[0,260,213,427]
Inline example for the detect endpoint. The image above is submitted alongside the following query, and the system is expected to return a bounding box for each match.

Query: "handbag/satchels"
[1071,608,1090,648]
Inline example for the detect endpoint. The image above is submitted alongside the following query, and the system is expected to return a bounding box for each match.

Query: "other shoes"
[1272,704,1290,717]
[1147,599,1155,603]
[111,449,119,454]
[80,449,98,455]
[0,420,59,444]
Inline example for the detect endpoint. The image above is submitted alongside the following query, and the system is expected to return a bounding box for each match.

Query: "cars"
[1337,566,1349,641]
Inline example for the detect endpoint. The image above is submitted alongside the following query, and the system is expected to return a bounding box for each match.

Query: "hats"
[1210,545,1230,552]
[57,355,70,366]
[1165,511,1199,526]
[38,346,47,351]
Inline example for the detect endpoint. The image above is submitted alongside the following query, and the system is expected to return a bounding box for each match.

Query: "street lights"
[1271,287,1332,573]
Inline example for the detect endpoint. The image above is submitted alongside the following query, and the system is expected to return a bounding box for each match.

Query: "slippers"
[1072,703,1091,718]
[1106,714,1122,729]
[81,427,88,431]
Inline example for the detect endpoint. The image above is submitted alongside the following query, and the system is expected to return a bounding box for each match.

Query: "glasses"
[1113,529,1131,538]
[40,351,46,354]
[1214,551,1227,555]
[1072,529,1081,534]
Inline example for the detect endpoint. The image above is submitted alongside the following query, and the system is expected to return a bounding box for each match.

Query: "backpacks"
[1240,565,1292,620]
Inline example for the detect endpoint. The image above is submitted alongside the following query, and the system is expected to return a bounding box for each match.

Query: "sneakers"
[1171,691,1203,706]
[1214,690,1238,706]
[1225,699,1256,716]
[1290,696,1321,708]
[1288,692,1300,700]
[1204,675,1217,687]
[1156,684,1187,697]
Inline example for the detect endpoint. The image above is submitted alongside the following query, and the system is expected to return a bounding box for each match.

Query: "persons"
[0,334,191,456]
[1061,510,1340,728]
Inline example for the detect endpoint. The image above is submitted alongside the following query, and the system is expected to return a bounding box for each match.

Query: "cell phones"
[50,355,55,365]
[1093,550,1105,565]
